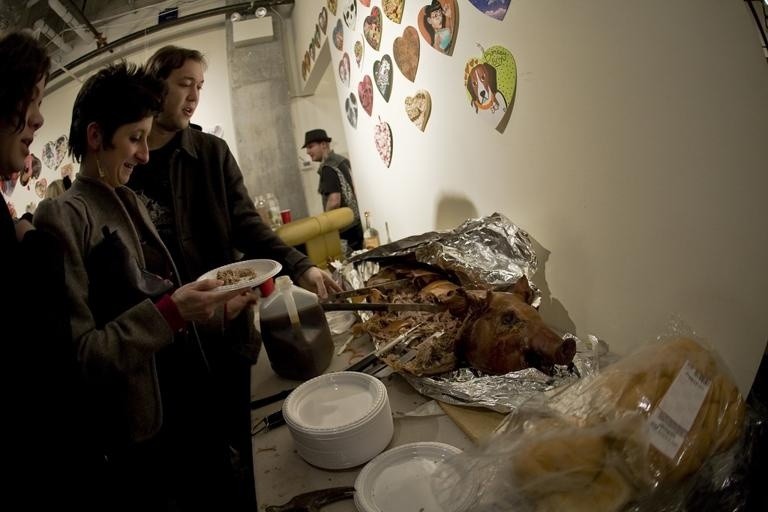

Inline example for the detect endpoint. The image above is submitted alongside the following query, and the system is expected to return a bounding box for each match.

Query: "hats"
[301,129,332,149]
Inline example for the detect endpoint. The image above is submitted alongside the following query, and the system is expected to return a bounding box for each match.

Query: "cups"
[279,208,292,224]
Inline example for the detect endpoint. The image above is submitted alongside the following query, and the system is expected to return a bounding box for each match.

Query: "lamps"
[227,6,275,49]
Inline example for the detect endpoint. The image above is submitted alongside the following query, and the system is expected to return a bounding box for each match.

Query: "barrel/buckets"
[259,276,334,382]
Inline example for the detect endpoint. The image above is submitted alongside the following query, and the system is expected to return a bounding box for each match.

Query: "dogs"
[466,62,500,113]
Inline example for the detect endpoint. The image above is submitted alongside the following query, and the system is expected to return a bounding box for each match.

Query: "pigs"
[457,273,577,375]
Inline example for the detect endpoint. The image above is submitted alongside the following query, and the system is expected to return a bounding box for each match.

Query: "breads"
[502,333,746,512]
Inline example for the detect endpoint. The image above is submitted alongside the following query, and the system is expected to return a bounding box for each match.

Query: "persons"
[35,60,266,511]
[300,128,362,250]
[424,2,454,49]
[0,28,53,509]
[146,46,344,434]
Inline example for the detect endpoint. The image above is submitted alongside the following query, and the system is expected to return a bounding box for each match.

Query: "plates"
[323,310,357,335]
[352,439,483,512]
[281,370,395,474]
[192,257,285,297]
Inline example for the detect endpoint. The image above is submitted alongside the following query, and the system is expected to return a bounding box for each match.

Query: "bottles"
[363,211,379,250]
[266,192,283,231]
[254,195,268,226]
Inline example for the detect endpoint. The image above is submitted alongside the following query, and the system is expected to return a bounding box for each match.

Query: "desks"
[271,203,355,271]
[244,286,542,511]
